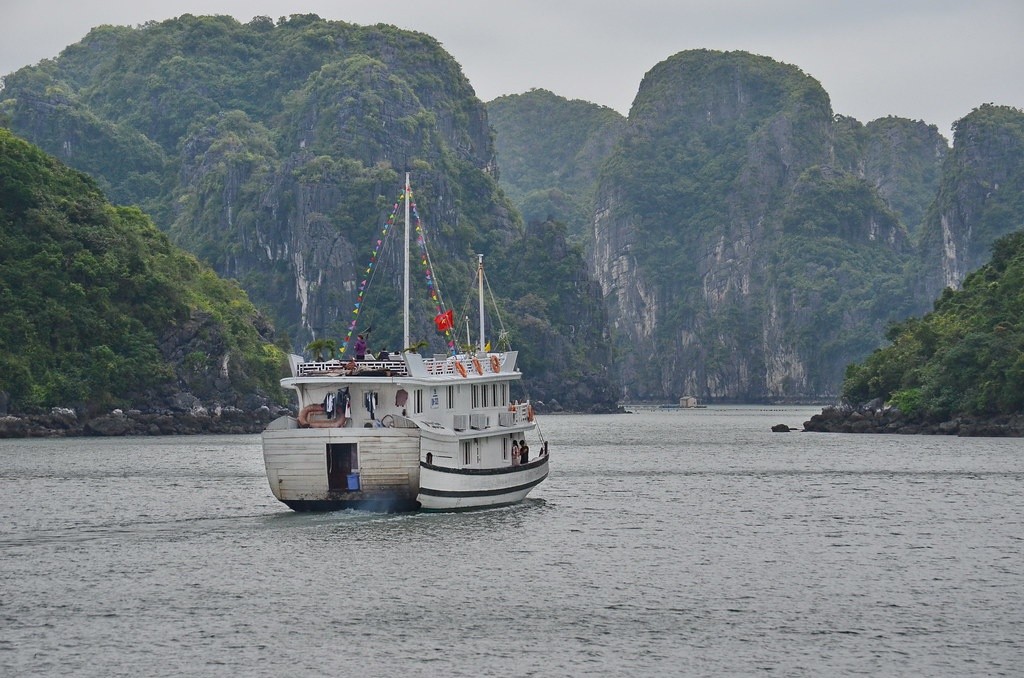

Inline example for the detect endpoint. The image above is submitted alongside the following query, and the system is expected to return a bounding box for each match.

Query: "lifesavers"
[473,357,483,375]
[490,355,500,373]
[455,361,467,377]
[528,404,534,421]
[509,406,516,411]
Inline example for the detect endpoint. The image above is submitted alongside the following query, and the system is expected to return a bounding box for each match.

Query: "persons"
[354,334,366,361]
[435,309,454,331]
[394,350,399,355]
[512,440,520,465]
[376,348,389,361]
[364,349,376,360]
[519,440,529,463]
[539,441,548,457]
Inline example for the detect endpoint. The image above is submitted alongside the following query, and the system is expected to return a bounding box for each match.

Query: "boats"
[297,403,349,429]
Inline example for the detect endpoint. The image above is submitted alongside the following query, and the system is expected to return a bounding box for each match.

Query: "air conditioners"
[498,413,514,427]
[471,414,486,428]
[453,415,468,430]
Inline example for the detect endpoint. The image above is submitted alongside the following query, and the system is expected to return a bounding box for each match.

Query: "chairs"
[478,351,487,359]
[433,354,448,361]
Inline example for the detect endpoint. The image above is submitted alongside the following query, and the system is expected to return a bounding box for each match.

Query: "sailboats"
[259,171,550,514]
